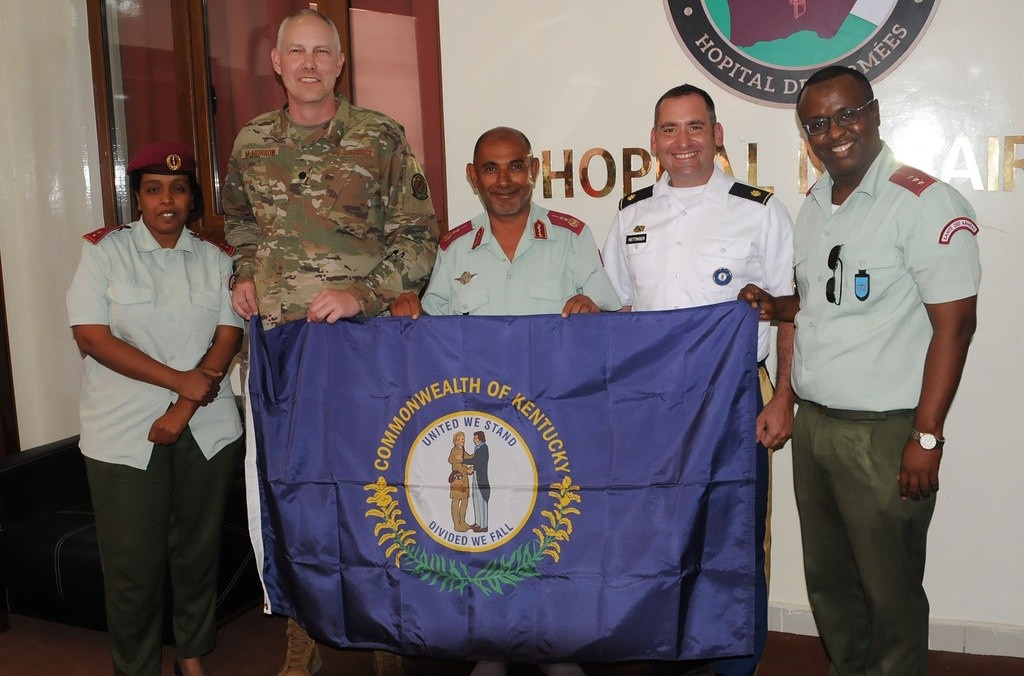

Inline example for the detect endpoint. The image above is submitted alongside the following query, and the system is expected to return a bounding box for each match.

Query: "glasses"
[803,98,873,135]
[826,243,843,306]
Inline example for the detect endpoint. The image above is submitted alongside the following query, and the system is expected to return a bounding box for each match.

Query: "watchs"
[909,428,946,450]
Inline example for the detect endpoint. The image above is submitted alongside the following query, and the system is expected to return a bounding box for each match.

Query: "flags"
[244,299,759,663]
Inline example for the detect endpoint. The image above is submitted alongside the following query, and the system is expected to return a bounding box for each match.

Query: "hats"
[126,141,196,178]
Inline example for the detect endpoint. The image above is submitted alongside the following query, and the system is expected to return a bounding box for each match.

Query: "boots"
[278,615,323,676]
[372,649,408,676]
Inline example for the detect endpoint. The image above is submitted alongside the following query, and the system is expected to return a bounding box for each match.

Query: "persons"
[64,142,246,676]
[600,85,795,676]
[219,8,440,325]
[389,127,622,319]
[738,66,981,676]
